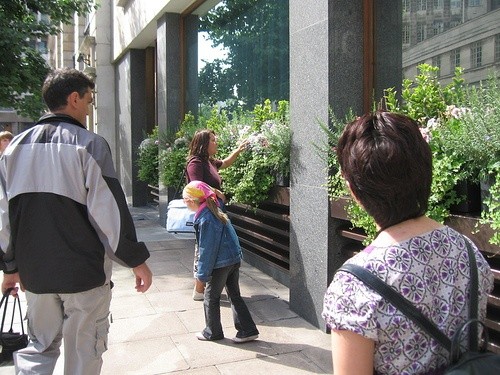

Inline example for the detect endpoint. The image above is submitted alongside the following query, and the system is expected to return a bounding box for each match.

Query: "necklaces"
[375,212,424,238]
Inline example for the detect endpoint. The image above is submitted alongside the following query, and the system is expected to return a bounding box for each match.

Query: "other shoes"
[196,332,224,340]
[193,288,206,300]
[233,334,258,342]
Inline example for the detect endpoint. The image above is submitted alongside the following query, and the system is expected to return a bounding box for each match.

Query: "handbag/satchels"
[166,199,196,234]
[0,287,29,367]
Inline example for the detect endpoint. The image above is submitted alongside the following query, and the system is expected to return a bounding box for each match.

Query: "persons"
[321,110,493,374]
[0,69,154,375]
[186,129,251,300]
[0,131,13,155]
[182,180,259,342]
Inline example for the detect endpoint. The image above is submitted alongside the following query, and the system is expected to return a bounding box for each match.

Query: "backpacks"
[334,236,500,375]
